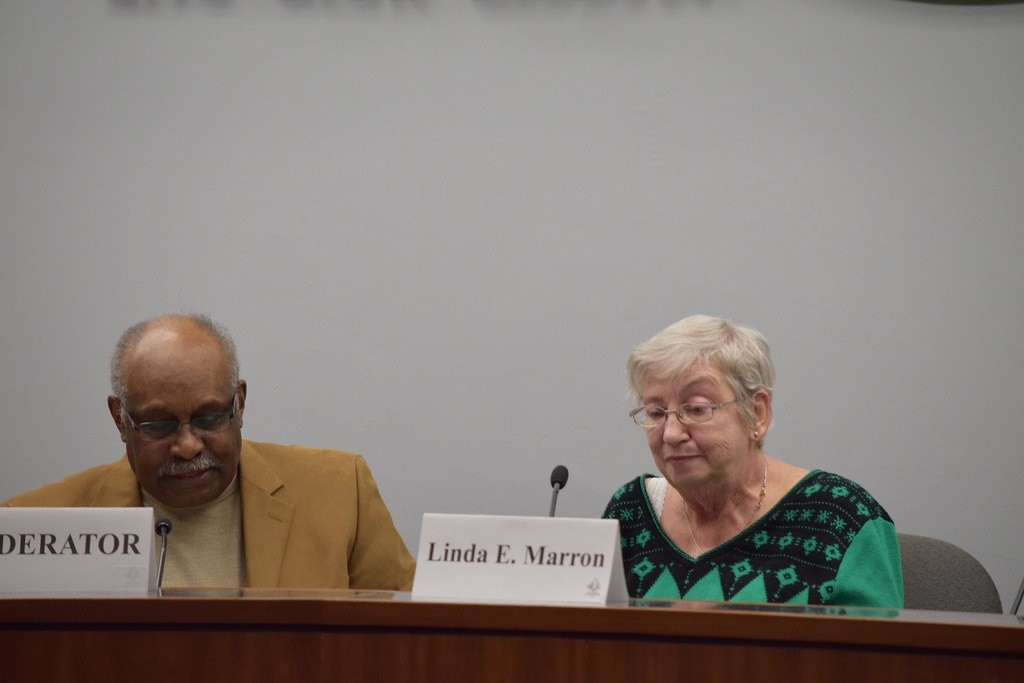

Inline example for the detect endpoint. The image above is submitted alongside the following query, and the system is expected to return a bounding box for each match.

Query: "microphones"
[154,517,173,586]
[550,465,569,518]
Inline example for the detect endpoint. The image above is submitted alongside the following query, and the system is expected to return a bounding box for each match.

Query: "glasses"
[630,399,737,427]
[122,394,236,441]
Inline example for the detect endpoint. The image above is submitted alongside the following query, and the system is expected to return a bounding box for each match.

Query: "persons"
[0,315,415,590]
[602,314,903,610]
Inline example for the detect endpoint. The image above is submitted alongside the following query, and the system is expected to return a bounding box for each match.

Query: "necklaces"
[682,464,768,555]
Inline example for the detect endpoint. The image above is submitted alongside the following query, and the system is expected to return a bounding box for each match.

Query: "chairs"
[896,532,1002,614]
